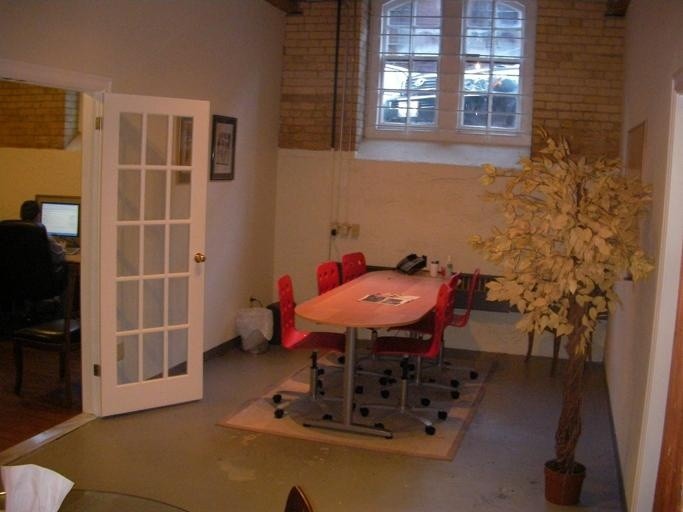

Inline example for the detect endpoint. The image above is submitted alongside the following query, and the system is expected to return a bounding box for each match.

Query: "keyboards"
[63,247,79,255]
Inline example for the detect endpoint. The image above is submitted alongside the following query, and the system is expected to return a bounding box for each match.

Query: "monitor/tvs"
[35,195,80,240]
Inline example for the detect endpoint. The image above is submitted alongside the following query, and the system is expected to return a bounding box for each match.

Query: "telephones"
[397,254,427,274]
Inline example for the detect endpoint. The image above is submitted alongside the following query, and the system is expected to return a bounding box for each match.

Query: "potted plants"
[465,125,655,505]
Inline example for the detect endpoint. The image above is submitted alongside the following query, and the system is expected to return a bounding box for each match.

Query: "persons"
[20,200,66,249]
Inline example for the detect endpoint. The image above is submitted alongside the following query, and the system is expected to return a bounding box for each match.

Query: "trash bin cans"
[236,307,270,355]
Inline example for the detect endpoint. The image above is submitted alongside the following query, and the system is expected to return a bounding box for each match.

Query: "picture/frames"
[174,114,237,185]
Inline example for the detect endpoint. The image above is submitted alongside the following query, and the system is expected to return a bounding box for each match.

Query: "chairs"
[270,253,482,440]
[0,219,79,408]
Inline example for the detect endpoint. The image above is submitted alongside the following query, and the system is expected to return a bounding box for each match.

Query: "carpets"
[214,348,498,462]
